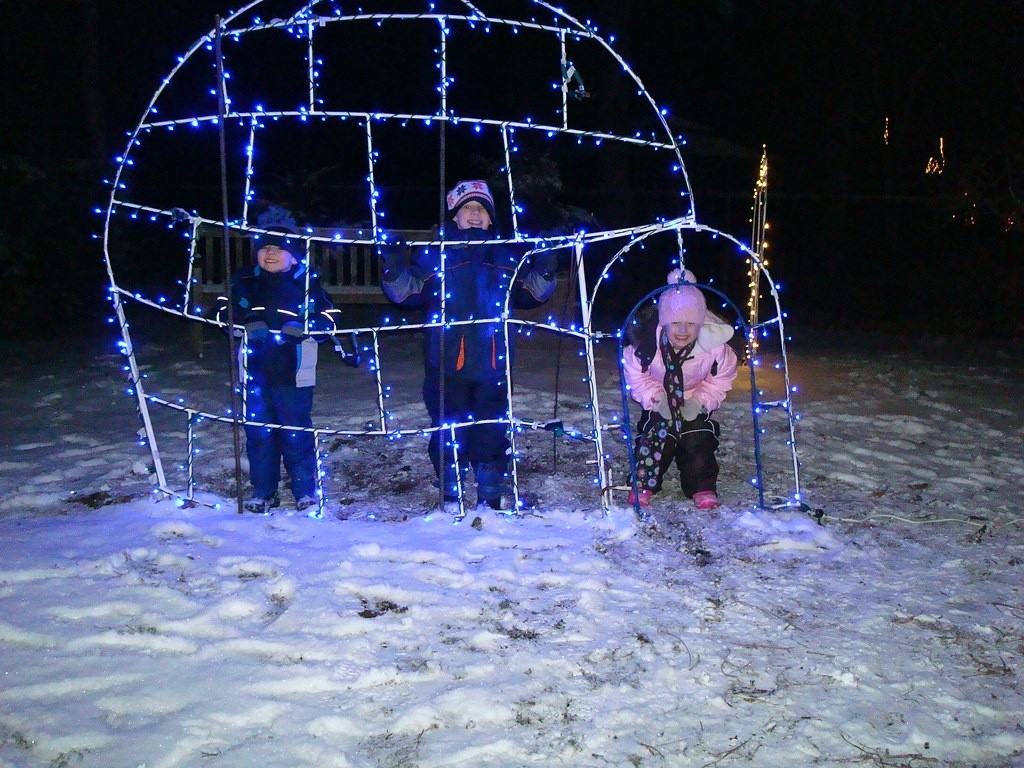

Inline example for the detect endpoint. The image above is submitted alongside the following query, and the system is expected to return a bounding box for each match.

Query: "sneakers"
[692,490,718,510]
[628,488,652,506]
[295,495,316,512]
[245,491,279,513]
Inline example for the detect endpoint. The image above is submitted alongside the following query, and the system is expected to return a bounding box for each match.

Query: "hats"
[254,227,302,264]
[445,179,497,228]
[658,269,706,327]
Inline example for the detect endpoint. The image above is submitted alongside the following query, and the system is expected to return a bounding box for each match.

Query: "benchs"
[189,223,435,358]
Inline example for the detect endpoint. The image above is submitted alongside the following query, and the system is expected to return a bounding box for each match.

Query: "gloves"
[376,235,407,281]
[280,321,305,343]
[533,231,559,280]
[680,398,702,421]
[653,395,672,420]
[245,320,269,342]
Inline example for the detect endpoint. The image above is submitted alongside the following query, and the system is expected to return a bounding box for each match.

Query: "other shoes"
[476,492,538,511]
[443,499,461,516]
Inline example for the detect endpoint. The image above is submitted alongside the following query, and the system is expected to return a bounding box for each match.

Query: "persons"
[622,269,738,508]
[214,206,337,515]
[377,179,561,513]
